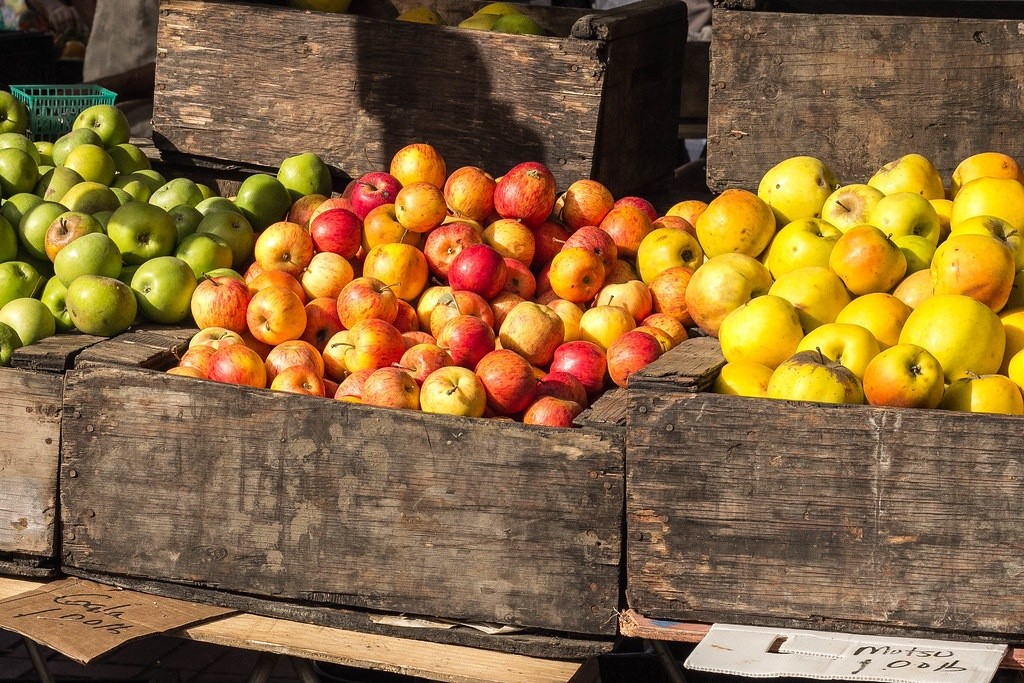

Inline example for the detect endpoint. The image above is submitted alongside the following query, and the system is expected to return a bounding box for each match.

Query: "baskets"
[7,84,117,142]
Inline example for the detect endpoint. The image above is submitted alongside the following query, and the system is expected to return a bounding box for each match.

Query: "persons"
[28,1,97,44]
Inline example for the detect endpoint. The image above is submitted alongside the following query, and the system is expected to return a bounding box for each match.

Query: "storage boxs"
[0,2,1024,639]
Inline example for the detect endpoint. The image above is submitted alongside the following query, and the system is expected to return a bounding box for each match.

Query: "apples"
[0,87,331,362]
[690,152,1023,415]
[302,0,547,38]
[163,144,708,428]
